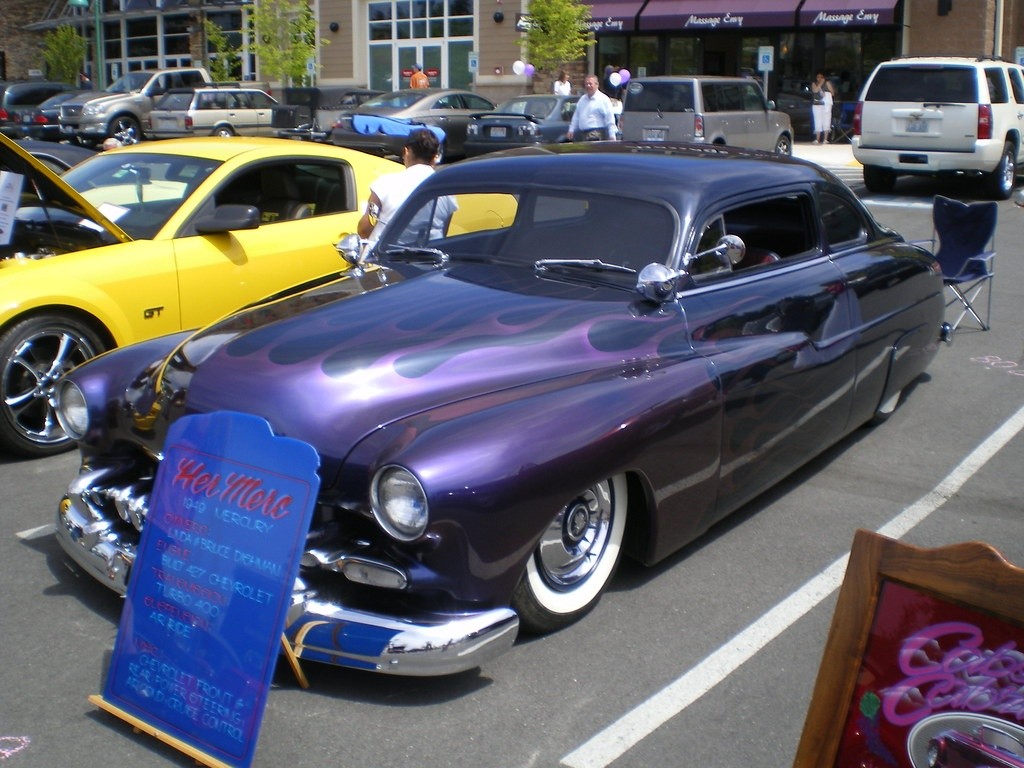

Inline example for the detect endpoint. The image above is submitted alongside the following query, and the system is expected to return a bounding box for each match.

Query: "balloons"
[606,64,630,88]
[513,61,535,76]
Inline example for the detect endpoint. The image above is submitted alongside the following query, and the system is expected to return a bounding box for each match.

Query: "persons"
[567,73,617,142]
[357,129,458,270]
[812,71,835,144]
[603,65,627,131]
[553,69,572,114]
[409,64,429,89]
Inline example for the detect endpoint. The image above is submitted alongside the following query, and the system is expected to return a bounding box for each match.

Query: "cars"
[0,129,527,456]
[7,140,136,225]
[144,87,284,141]
[330,87,494,166]
[1,78,105,149]
[464,92,583,152]
[619,75,794,157]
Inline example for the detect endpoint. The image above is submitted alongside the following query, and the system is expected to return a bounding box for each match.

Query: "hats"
[412,64,422,70]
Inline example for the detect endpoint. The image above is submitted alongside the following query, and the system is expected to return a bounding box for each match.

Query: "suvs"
[270,83,386,142]
[852,55,1024,201]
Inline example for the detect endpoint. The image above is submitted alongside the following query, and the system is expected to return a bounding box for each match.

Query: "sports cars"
[55,140,962,677]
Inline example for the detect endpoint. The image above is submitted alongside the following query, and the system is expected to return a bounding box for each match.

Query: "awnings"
[548,0,902,33]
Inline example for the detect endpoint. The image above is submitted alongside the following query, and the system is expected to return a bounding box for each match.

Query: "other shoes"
[812,140,819,144]
[822,139,829,144]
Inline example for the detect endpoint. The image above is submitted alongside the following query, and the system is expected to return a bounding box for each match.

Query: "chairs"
[834,102,856,143]
[250,163,312,224]
[912,195,1000,341]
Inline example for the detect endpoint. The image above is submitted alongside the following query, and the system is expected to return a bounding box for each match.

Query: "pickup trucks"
[58,66,275,148]
